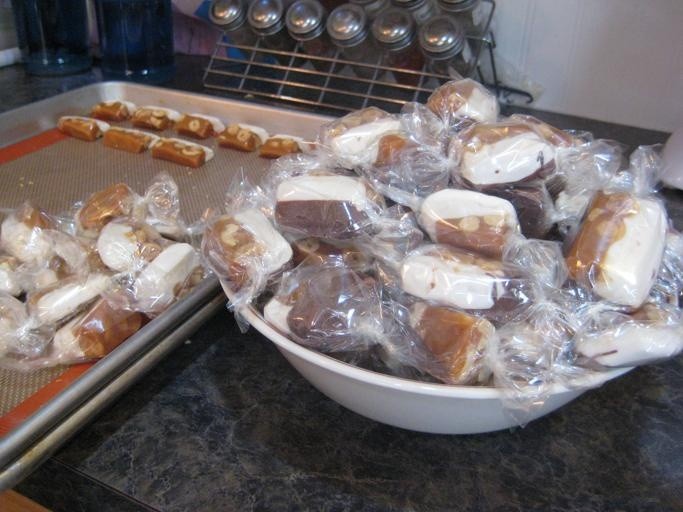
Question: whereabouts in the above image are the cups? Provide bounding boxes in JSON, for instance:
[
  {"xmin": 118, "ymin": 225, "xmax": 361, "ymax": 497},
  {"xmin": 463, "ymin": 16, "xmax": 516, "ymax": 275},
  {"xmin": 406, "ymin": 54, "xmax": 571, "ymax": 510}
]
[{"xmin": 11, "ymin": 0, "xmax": 175, "ymax": 78}]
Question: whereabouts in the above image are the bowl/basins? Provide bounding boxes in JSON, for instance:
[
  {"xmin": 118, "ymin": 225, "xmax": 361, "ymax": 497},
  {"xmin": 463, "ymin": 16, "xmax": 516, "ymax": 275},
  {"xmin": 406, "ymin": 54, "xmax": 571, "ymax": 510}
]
[{"xmin": 217, "ymin": 260, "xmax": 679, "ymax": 435}]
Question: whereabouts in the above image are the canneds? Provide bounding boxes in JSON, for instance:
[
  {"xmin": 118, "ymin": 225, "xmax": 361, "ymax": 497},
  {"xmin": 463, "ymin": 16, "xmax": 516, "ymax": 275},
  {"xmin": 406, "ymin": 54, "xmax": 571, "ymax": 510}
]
[{"xmin": 207, "ymin": 0, "xmax": 484, "ymax": 86}]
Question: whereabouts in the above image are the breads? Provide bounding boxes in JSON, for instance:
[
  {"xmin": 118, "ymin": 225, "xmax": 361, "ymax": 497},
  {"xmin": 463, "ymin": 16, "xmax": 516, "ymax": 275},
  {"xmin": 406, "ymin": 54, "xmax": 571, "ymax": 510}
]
[{"xmin": 0, "ymin": 78, "xmax": 668, "ymax": 384}]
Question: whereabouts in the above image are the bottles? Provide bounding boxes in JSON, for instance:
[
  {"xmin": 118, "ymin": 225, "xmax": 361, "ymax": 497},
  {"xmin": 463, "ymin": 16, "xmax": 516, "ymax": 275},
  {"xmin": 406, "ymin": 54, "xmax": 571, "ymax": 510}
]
[{"xmin": 209, "ymin": 0, "xmax": 479, "ymax": 89}]
